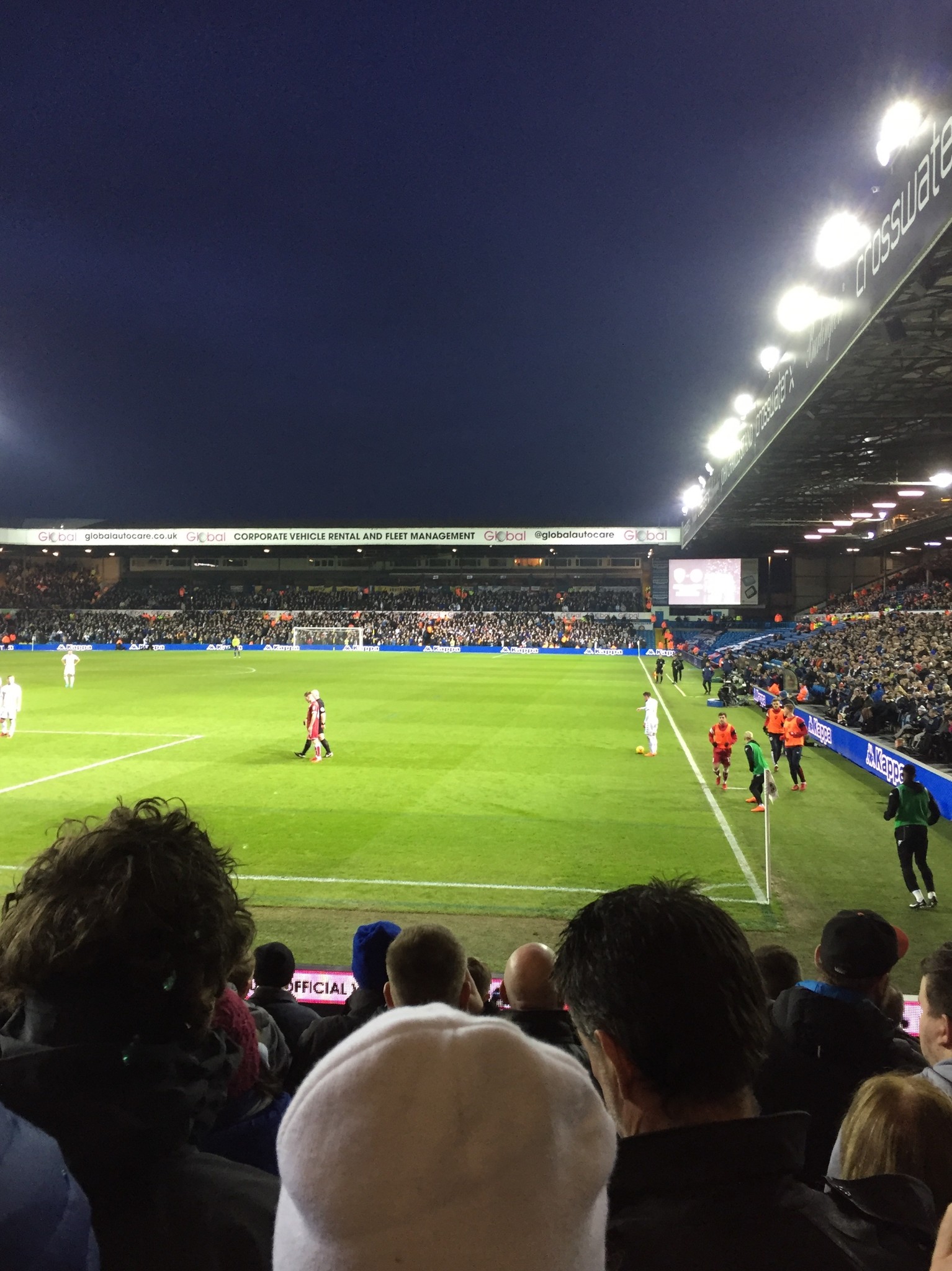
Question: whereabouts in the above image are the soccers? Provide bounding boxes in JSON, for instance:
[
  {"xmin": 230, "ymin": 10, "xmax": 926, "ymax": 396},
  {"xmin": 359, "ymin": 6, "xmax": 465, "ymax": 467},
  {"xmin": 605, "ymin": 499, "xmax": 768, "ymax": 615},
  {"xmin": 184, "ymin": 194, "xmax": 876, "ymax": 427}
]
[{"xmin": 636, "ymin": 745, "xmax": 644, "ymax": 754}]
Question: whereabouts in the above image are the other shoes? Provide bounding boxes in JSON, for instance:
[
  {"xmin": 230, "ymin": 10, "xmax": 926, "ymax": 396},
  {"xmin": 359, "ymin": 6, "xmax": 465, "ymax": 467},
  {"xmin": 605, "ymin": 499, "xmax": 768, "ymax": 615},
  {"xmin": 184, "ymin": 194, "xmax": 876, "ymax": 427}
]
[
  {"xmin": 705, "ymin": 691, "xmax": 708, "ymax": 694},
  {"xmin": 66, "ymin": 684, "xmax": 73, "ymax": 688},
  {"xmin": 1, "ymin": 732, "xmax": 14, "ymax": 739},
  {"xmin": 655, "ymin": 680, "xmax": 682, "ymax": 684},
  {"xmin": 645, "ymin": 753, "xmax": 657, "ymax": 757},
  {"xmin": 238, "ymin": 656, "xmax": 241, "ymax": 658},
  {"xmin": 234, "ymin": 656, "xmax": 236, "ymax": 658},
  {"xmin": 708, "ymin": 692, "xmax": 710, "ymax": 694}
]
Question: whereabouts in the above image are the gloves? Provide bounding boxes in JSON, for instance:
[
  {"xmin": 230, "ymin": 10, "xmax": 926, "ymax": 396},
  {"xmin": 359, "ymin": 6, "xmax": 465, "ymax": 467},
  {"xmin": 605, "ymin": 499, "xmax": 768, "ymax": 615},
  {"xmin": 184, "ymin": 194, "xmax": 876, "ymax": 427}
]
[
  {"xmin": 712, "ymin": 742, "xmax": 717, "ymax": 747},
  {"xmin": 779, "ymin": 736, "xmax": 784, "ymax": 741},
  {"xmin": 724, "ymin": 742, "xmax": 729, "ymax": 747},
  {"xmin": 763, "ymin": 726, "xmax": 767, "ymax": 731},
  {"xmin": 750, "ymin": 769, "xmax": 754, "ymax": 772},
  {"xmin": 789, "ymin": 731, "xmax": 796, "ymax": 737}
]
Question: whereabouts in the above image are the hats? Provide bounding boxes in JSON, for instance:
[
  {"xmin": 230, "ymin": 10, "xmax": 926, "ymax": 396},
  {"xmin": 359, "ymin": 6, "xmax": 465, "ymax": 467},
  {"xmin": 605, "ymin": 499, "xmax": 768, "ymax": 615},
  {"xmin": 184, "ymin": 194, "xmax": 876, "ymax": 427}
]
[
  {"xmin": 820, "ymin": 908, "xmax": 908, "ymax": 980},
  {"xmin": 918, "ymin": 705, "xmax": 926, "ymax": 711},
  {"xmin": 269, "ymin": 1003, "xmax": 617, "ymax": 1270},
  {"xmin": 351, "ymin": 922, "xmax": 401, "ymax": 990},
  {"xmin": 253, "ymin": 942, "xmax": 296, "ymax": 987}
]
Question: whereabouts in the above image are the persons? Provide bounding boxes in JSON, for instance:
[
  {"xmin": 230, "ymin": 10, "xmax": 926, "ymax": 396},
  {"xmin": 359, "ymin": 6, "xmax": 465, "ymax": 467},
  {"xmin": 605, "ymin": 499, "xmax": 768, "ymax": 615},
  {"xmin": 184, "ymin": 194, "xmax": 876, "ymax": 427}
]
[
  {"xmin": 709, "ymin": 713, "xmax": 737, "ymax": 789},
  {"xmin": 745, "ymin": 731, "xmax": 769, "ymax": 811},
  {"xmin": 779, "ymin": 704, "xmax": 808, "ymax": 791},
  {"xmin": 0, "ymin": 676, "xmax": 22, "ymax": 737},
  {"xmin": 0, "ymin": 559, "xmax": 657, "ymax": 659},
  {"xmin": 294, "ymin": 689, "xmax": 333, "ymax": 761},
  {"xmin": 884, "ymin": 763, "xmax": 940, "ymax": 908},
  {"xmin": 61, "ymin": 650, "xmax": 80, "ymax": 688},
  {"xmin": 636, "ymin": 692, "xmax": 659, "ymax": 756},
  {"xmin": 655, "ymin": 555, "xmax": 952, "ymax": 773},
  {"xmin": 0, "ymin": 798, "xmax": 952, "ymax": 1271}
]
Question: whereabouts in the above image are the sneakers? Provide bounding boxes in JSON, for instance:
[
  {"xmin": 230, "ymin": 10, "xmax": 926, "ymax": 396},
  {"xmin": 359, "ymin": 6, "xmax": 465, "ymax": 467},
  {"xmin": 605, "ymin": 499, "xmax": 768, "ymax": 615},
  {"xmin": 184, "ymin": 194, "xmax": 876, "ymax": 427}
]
[
  {"xmin": 715, "ymin": 776, "xmax": 764, "ymax": 812},
  {"xmin": 791, "ymin": 785, "xmax": 799, "ymax": 791},
  {"xmin": 909, "ymin": 899, "xmax": 926, "ymax": 909},
  {"xmin": 800, "ymin": 783, "xmax": 806, "ymax": 791},
  {"xmin": 774, "ymin": 766, "xmax": 779, "ymax": 772},
  {"xmin": 926, "ymin": 896, "xmax": 938, "ymax": 908},
  {"xmin": 294, "ymin": 751, "xmax": 334, "ymax": 762}
]
